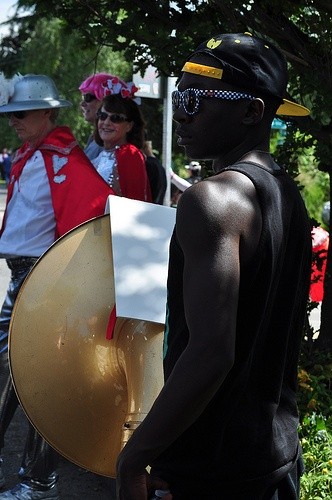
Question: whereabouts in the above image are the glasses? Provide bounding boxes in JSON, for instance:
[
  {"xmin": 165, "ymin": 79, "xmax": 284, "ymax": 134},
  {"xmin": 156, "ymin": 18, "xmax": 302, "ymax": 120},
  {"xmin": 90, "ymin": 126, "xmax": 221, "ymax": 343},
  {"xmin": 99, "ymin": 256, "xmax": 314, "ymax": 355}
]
[
  {"xmin": 172, "ymin": 88, "xmax": 255, "ymax": 116},
  {"xmin": 96, "ymin": 111, "xmax": 128, "ymax": 123},
  {"xmin": 82, "ymin": 93, "xmax": 96, "ymax": 102},
  {"xmin": 6, "ymin": 111, "xmax": 38, "ymax": 119}
]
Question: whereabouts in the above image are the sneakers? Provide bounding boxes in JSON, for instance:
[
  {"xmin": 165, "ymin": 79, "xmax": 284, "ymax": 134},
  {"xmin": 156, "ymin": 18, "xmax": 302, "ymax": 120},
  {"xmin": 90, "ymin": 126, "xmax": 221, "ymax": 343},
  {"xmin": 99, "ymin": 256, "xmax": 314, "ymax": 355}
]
[{"xmin": 0, "ymin": 473, "xmax": 59, "ymax": 500}]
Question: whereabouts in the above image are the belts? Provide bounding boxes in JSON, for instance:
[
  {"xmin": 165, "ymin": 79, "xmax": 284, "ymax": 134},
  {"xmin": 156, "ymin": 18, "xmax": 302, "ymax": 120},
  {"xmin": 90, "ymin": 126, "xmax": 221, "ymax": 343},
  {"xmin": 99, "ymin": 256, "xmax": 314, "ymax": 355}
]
[{"xmin": 6, "ymin": 258, "xmax": 37, "ymax": 269}]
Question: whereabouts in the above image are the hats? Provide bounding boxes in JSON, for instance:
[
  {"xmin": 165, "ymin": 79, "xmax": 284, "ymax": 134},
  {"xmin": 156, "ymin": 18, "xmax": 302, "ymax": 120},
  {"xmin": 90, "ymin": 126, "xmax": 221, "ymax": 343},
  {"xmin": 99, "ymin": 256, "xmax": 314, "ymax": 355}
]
[
  {"xmin": 0, "ymin": 74, "xmax": 72, "ymax": 113},
  {"xmin": 181, "ymin": 32, "xmax": 311, "ymax": 116},
  {"xmin": 184, "ymin": 161, "xmax": 201, "ymax": 170}
]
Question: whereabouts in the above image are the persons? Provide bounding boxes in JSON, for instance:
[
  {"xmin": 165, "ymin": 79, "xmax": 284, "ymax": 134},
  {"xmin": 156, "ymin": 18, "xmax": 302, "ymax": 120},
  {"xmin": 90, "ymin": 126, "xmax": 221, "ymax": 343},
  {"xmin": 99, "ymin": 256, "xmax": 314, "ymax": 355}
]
[
  {"xmin": 113, "ymin": 34, "xmax": 312, "ymax": 500},
  {"xmin": 0, "ymin": 74, "xmax": 332, "ymax": 500}
]
